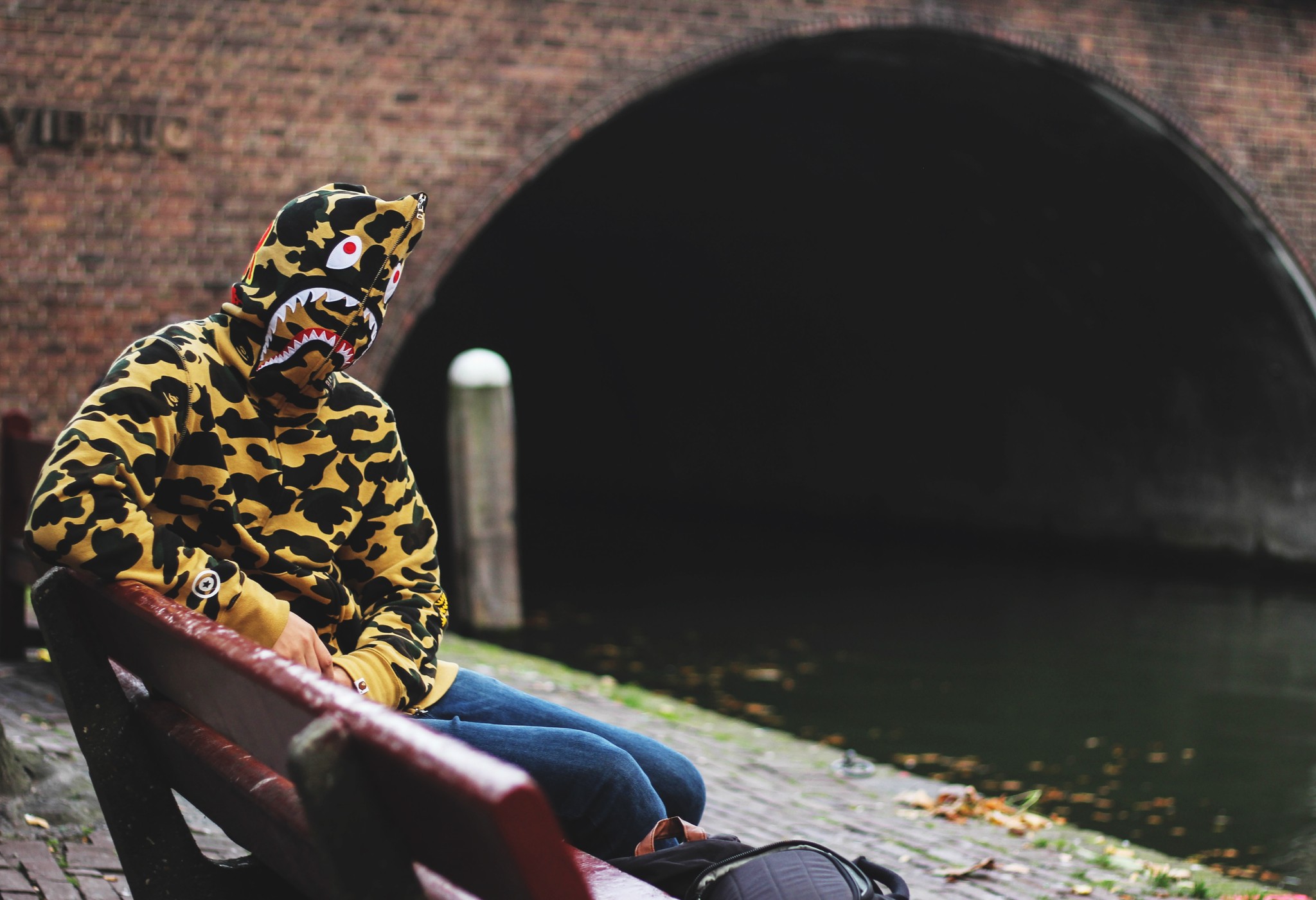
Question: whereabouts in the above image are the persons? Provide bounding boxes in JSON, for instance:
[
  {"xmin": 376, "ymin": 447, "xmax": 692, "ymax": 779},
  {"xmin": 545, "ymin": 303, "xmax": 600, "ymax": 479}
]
[{"xmin": 23, "ymin": 182, "xmax": 710, "ymax": 866}]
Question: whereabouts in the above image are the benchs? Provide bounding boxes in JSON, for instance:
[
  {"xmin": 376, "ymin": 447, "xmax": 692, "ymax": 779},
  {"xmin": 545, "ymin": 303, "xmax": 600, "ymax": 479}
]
[{"xmin": 26, "ymin": 569, "xmax": 688, "ymax": 899}]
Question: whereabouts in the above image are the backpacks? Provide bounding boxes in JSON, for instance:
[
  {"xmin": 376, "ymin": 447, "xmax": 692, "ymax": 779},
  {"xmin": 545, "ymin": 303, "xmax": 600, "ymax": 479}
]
[{"xmin": 606, "ymin": 816, "xmax": 910, "ymax": 900}]
